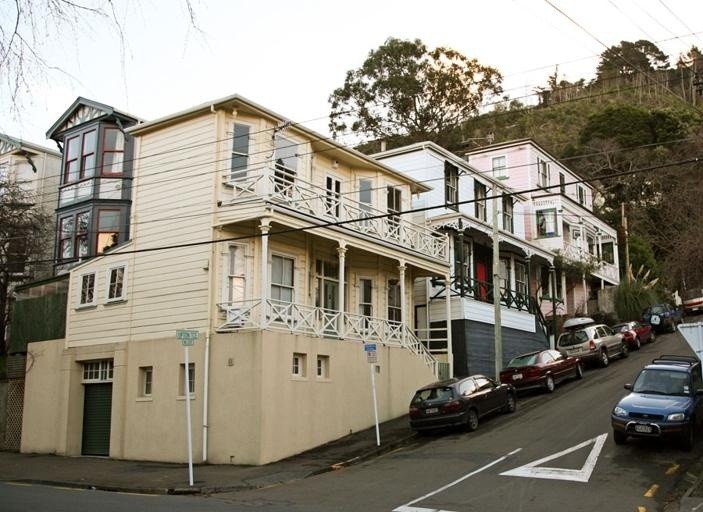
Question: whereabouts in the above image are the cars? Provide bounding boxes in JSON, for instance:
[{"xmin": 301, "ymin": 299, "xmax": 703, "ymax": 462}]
[
  {"xmin": 498, "ymin": 349, "xmax": 585, "ymax": 394},
  {"xmin": 406, "ymin": 374, "xmax": 519, "ymax": 433},
  {"xmin": 611, "ymin": 321, "xmax": 655, "ymax": 349}
]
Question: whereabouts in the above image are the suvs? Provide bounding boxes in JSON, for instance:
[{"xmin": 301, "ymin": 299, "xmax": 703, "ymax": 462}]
[
  {"xmin": 611, "ymin": 355, "xmax": 702, "ymax": 451},
  {"xmin": 557, "ymin": 316, "xmax": 628, "ymax": 368},
  {"xmin": 640, "ymin": 303, "xmax": 684, "ymax": 334}
]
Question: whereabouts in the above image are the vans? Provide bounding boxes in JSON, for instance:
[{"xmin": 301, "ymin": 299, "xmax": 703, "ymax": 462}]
[{"xmin": 682, "ymin": 288, "xmax": 702, "ymax": 316}]
